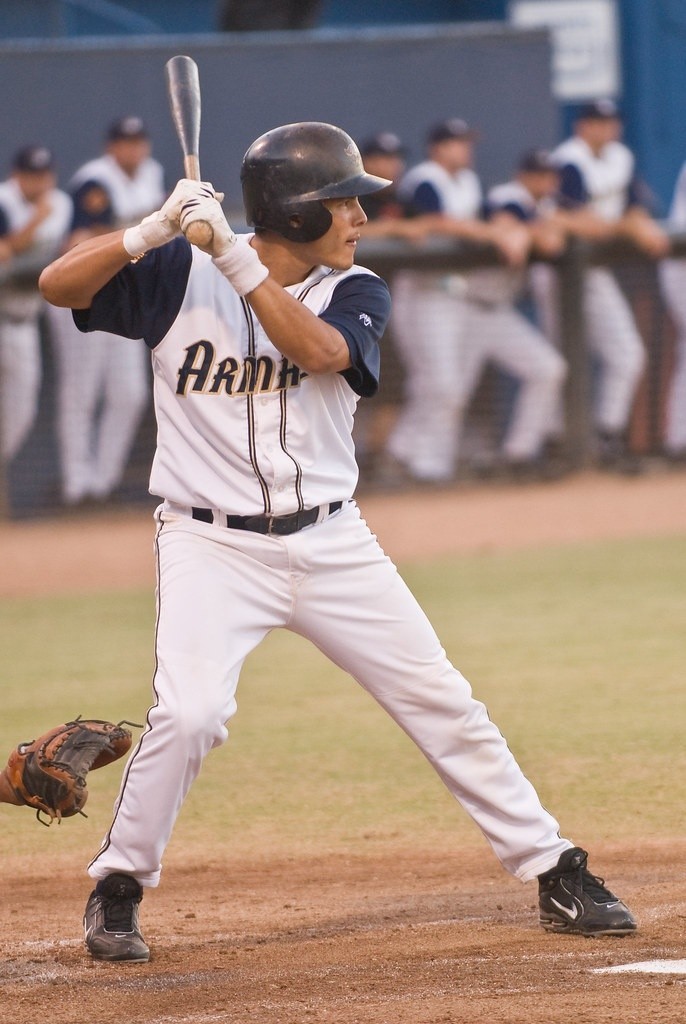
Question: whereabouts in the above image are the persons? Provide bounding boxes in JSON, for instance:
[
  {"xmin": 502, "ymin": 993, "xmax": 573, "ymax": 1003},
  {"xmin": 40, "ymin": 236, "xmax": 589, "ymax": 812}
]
[
  {"xmin": 0, "ymin": 92, "xmax": 686, "ymax": 522},
  {"xmin": 0, "ymin": 768, "xmax": 25, "ymax": 807},
  {"xmin": 38, "ymin": 121, "xmax": 639, "ymax": 960}
]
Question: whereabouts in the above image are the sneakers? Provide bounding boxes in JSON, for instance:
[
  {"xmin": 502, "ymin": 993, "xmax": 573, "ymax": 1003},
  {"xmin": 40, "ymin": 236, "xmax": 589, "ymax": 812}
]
[
  {"xmin": 537, "ymin": 846, "xmax": 638, "ymax": 938},
  {"xmin": 82, "ymin": 872, "xmax": 151, "ymax": 963}
]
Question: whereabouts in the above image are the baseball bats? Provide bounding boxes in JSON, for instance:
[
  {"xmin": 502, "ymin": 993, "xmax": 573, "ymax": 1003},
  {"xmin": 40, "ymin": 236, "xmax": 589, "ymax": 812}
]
[{"xmin": 166, "ymin": 53, "xmax": 216, "ymax": 251}]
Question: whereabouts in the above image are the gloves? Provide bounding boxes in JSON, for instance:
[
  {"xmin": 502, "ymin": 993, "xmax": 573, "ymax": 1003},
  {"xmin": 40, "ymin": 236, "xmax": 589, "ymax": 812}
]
[
  {"xmin": 122, "ymin": 178, "xmax": 225, "ymax": 257},
  {"xmin": 178, "ymin": 195, "xmax": 270, "ymax": 296}
]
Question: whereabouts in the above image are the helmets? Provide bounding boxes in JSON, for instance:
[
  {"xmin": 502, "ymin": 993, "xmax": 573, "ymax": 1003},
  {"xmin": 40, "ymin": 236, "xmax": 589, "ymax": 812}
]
[{"xmin": 240, "ymin": 121, "xmax": 394, "ymax": 243}]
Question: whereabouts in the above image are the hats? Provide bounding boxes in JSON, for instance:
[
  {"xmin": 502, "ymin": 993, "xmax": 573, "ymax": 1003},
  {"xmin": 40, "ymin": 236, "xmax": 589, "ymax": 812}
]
[
  {"xmin": 107, "ymin": 116, "xmax": 151, "ymax": 140},
  {"xmin": 360, "ymin": 128, "xmax": 410, "ymax": 159},
  {"xmin": 523, "ymin": 148, "xmax": 568, "ymax": 172},
  {"xmin": 574, "ymin": 97, "xmax": 625, "ymax": 120},
  {"xmin": 425, "ymin": 116, "xmax": 479, "ymax": 144},
  {"xmin": 13, "ymin": 143, "xmax": 59, "ymax": 174}
]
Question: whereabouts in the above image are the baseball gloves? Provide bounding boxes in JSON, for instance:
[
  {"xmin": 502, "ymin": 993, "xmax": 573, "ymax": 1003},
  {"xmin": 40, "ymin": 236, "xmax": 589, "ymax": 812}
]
[{"xmin": 6, "ymin": 718, "xmax": 133, "ymax": 823}]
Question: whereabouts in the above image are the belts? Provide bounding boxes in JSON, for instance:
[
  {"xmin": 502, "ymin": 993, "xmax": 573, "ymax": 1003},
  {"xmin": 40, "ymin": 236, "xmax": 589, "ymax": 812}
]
[
  {"xmin": 0, "ymin": 311, "xmax": 26, "ymax": 324},
  {"xmin": 192, "ymin": 499, "xmax": 352, "ymax": 535}
]
